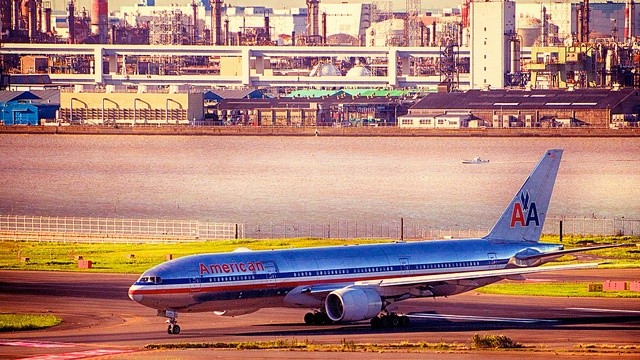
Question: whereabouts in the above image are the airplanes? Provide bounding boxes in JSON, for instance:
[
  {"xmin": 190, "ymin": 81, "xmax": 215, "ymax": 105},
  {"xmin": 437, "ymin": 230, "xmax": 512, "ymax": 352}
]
[{"xmin": 128, "ymin": 149, "xmax": 635, "ymax": 333}]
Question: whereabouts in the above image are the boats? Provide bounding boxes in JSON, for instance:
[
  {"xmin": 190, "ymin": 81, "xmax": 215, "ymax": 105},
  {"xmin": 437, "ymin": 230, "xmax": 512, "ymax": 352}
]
[{"xmin": 461, "ymin": 159, "xmax": 489, "ymax": 163}]
